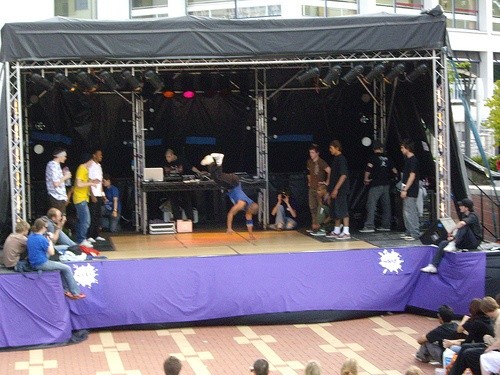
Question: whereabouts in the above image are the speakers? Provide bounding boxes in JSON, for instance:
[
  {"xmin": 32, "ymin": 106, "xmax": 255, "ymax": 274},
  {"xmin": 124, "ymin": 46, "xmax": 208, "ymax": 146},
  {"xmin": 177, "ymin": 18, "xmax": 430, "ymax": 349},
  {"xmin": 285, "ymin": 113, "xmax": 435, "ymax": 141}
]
[{"xmin": 419, "ymin": 217, "xmax": 455, "ymax": 245}]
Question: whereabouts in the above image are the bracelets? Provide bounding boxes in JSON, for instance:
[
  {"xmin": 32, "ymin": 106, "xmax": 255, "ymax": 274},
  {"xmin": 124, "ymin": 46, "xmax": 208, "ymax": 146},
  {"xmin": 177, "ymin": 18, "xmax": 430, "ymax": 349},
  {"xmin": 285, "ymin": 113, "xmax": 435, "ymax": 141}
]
[
  {"xmin": 113, "ymin": 209, "xmax": 117, "ymax": 212},
  {"xmin": 402, "ymin": 189, "xmax": 407, "ymax": 192}
]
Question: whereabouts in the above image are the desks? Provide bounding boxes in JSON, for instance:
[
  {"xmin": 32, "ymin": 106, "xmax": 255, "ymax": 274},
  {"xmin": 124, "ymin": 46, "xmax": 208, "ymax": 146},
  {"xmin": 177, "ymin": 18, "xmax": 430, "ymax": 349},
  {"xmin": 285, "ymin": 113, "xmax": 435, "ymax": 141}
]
[{"xmin": 140, "ymin": 177, "xmax": 267, "ymax": 236}]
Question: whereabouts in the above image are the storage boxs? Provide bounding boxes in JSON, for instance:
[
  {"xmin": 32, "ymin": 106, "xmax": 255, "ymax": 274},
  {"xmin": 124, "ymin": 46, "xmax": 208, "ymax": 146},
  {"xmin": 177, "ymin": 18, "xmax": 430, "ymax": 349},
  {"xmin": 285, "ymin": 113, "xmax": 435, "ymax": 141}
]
[
  {"xmin": 442, "ymin": 347, "xmax": 456, "ymax": 369},
  {"xmin": 149, "ymin": 220, "xmax": 176, "ymax": 235},
  {"xmin": 176, "ymin": 219, "xmax": 193, "ymax": 233}
]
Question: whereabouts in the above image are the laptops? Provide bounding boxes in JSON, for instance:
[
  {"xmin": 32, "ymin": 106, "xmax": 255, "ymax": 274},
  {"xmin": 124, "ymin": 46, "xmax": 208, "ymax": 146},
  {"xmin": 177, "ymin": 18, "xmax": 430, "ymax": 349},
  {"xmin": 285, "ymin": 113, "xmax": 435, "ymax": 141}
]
[{"xmin": 143, "ymin": 168, "xmax": 164, "ymax": 181}]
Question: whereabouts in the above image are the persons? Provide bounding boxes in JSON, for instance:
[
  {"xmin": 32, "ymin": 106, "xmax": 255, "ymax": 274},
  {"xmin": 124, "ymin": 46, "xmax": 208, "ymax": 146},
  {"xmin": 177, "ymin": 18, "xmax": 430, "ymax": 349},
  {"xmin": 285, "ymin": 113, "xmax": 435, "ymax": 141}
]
[
  {"xmin": 162, "ymin": 296, "xmax": 500, "ymax": 375},
  {"xmin": 1, "ymin": 139, "xmax": 420, "ymax": 300},
  {"xmin": 420, "ymin": 199, "xmax": 482, "ymax": 273}
]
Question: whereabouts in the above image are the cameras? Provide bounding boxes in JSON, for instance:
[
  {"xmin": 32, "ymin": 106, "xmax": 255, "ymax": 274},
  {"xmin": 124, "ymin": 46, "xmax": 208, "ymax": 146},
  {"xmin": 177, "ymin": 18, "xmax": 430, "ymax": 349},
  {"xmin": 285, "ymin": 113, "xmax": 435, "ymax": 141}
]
[{"xmin": 281, "ymin": 194, "xmax": 285, "ymax": 199}]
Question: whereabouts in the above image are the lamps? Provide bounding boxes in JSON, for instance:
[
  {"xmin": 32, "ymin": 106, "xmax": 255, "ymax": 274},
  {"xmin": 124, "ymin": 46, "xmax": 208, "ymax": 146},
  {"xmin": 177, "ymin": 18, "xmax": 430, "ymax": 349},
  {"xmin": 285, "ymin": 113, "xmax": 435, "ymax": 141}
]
[
  {"xmin": 203, "ymin": 73, "xmax": 218, "ymax": 99},
  {"xmin": 99, "ymin": 70, "xmax": 120, "ymax": 92},
  {"xmin": 362, "ymin": 63, "xmax": 385, "ymax": 86},
  {"xmin": 294, "ymin": 65, "xmax": 319, "ymax": 87},
  {"xmin": 161, "ymin": 74, "xmax": 175, "ymax": 98},
  {"xmin": 219, "ymin": 72, "xmax": 234, "ymax": 96},
  {"xmin": 341, "ymin": 65, "xmax": 365, "ymax": 87},
  {"xmin": 144, "ymin": 70, "xmax": 164, "ymax": 94},
  {"xmin": 51, "ymin": 72, "xmax": 76, "ymax": 95},
  {"xmin": 382, "ymin": 63, "xmax": 405, "ymax": 85},
  {"xmin": 182, "ymin": 75, "xmax": 195, "ymax": 99},
  {"xmin": 76, "ymin": 71, "xmax": 99, "ymax": 93},
  {"xmin": 119, "ymin": 70, "xmax": 142, "ymax": 92},
  {"xmin": 403, "ymin": 63, "xmax": 429, "ymax": 84},
  {"xmin": 322, "ymin": 65, "xmax": 342, "ymax": 88},
  {"xmin": 238, "ymin": 68, "xmax": 252, "ymax": 96},
  {"xmin": 29, "ymin": 72, "xmax": 55, "ymax": 93}
]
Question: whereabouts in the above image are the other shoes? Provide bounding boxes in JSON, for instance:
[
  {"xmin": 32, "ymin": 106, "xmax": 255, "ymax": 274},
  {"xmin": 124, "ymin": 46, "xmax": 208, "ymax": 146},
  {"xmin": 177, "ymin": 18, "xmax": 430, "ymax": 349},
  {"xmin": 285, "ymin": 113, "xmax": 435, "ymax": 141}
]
[
  {"xmin": 358, "ymin": 227, "xmax": 375, "ymax": 232},
  {"xmin": 399, "ymin": 233, "xmax": 417, "ymax": 240},
  {"xmin": 335, "ymin": 231, "xmax": 351, "ymax": 240},
  {"xmin": 88, "ymin": 237, "xmax": 96, "ymax": 243},
  {"xmin": 442, "ymin": 241, "xmax": 456, "ymax": 252},
  {"xmin": 309, "ymin": 230, "xmax": 326, "ymax": 236},
  {"xmin": 200, "ymin": 155, "xmax": 214, "ymax": 166},
  {"xmin": 64, "ymin": 292, "xmax": 87, "ymax": 300},
  {"xmin": 211, "ymin": 152, "xmax": 225, "ymax": 164},
  {"xmin": 325, "ymin": 231, "xmax": 341, "ymax": 238},
  {"xmin": 375, "ymin": 226, "xmax": 391, "ymax": 231},
  {"xmin": 416, "ymin": 350, "xmax": 431, "ymax": 363},
  {"xmin": 420, "ymin": 264, "xmax": 438, "ymax": 273},
  {"xmin": 80, "ymin": 240, "xmax": 93, "ymax": 248},
  {"xmin": 96, "ymin": 236, "xmax": 106, "ymax": 240},
  {"xmin": 306, "ymin": 229, "xmax": 314, "ymax": 233}
]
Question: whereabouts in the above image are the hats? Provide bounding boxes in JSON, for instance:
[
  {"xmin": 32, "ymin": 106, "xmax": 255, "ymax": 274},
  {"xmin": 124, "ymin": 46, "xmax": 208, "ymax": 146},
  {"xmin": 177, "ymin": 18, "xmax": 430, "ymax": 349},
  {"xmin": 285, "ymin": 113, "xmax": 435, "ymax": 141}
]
[
  {"xmin": 457, "ymin": 198, "xmax": 473, "ymax": 208},
  {"xmin": 440, "ymin": 305, "xmax": 453, "ymax": 320},
  {"xmin": 53, "ymin": 148, "xmax": 66, "ymax": 157},
  {"xmin": 331, "ymin": 139, "xmax": 343, "ymax": 151}
]
